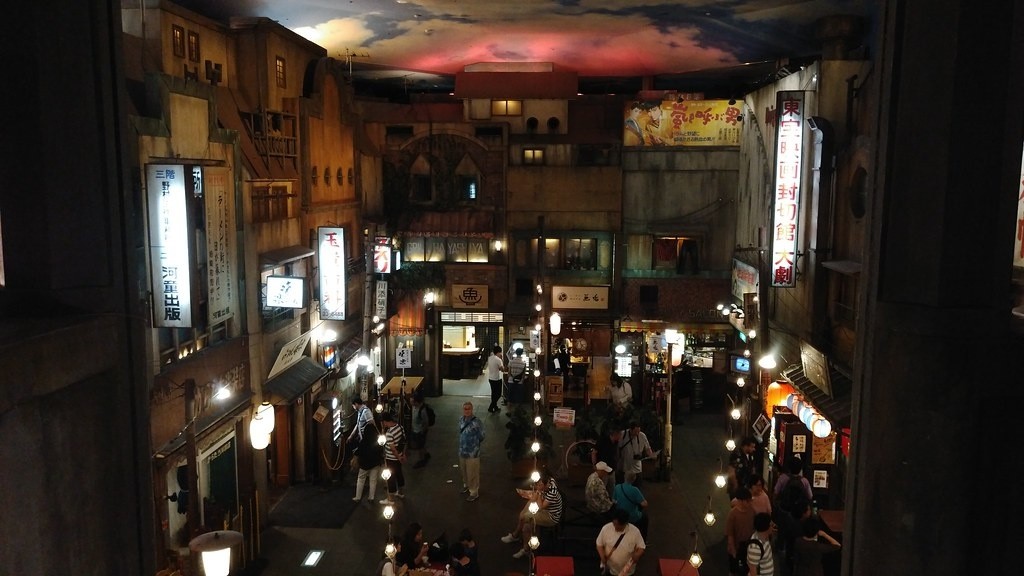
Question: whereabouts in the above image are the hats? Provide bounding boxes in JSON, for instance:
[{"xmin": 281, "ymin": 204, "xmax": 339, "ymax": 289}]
[{"xmin": 596, "ymin": 461, "xmax": 613, "ymax": 472}]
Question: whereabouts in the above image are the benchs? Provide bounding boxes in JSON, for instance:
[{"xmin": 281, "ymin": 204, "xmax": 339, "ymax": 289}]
[{"xmin": 534, "ymin": 490, "xmax": 613, "ymax": 543}]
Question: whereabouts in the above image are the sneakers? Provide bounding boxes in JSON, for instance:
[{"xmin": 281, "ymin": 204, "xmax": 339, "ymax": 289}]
[
  {"xmin": 513, "ymin": 549, "xmax": 530, "ymax": 559},
  {"xmin": 501, "ymin": 533, "xmax": 519, "ymax": 543}
]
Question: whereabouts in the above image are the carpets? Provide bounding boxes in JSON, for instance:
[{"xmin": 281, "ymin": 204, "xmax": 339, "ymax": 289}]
[{"xmin": 268, "ymin": 477, "xmax": 369, "ymax": 529}]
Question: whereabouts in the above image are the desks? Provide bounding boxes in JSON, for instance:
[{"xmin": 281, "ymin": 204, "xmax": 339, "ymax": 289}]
[
  {"xmin": 533, "ymin": 556, "xmax": 575, "ymax": 576},
  {"xmin": 380, "ymin": 374, "xmax": 425, "ymax": 411},
  {"xmin": 817, "ymin": 509, "xmax": 845, "ymax": 533},
  {"xmin": 411, "ymin": 560, "xmax": 456, "ymax": 576},
  {"xmin": 658, "ymin": 556, "xmax": 703, "ymax": 575}
]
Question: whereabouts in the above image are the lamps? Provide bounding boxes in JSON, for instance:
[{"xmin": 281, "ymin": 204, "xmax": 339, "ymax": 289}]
[
  {"xmin": 728, "ymin": 95, "xmax": 737, "ymax": 106},
  {"xmin": 737, "ymin": 113, "xmax": 745, "ymax": 122},
  {"xmin": 677, "ymin": 94, "xmax": 684, "ymax": 104}
]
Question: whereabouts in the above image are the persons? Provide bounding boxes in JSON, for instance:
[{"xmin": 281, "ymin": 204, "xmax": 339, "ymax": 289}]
[
  {"xmin": 381, "ymin": 413, "xmax": 408, "ymax": 499},
  {"xmin": 376, "ymin": 523, "xmax": 480, "ymax": 576},
  {"xmin": 501, "ymin": 469, "xmax": 562, "ymax": 559},
  {"xmin": 785, "ymin": 500, "xmax": 811, "ymax": 566},
  {"xmin": 483, "ymin": 346, "xmax": 504, "ymax": 413},
  {"xmin": 504, "ymin": 407, "xmax": 532, "ymax": 470},
  {"xmin": 559, "ymin": 345, "xmax": 570, "ymax": 391},
  {"xmin": 596, "ymin": 509, "xmax": 646, "ymax": 576},
  {"xmin": 791, "ymin": 519, "xmax": 841, "ymax": 576},
  {"xmin": 506, "ymin": 348, "xmax": 527, "ymax": 417},
  {"xmin": 352, "ymin": 423, "xmax": 385, "ymax": 500},
  {"xmin": 458, "ymin": 403, "xmax": 485, "ymax": 501},
  {"xmin": 570, "ymin": 422, "xmax": 657, "ymax": 548},
  {"xmin": 727, "ymin": 488, "xmax": 777, "ymax": 576},
  {"xmin": 410, "ymin": 394, "xmax": 428, "ymax": 468},
  {"xmin": 609, "ymin": 373, "xmax": 633, "ymax": 417},
  {"xmin": 773, "ymin": 457, "xmax": 813, "ymax": 552},
  {"xmin": 727, "ymin": 438, "xmax": 755, "ymax": 501},
  {"xmin": 345, "ymin": 398, "xmax": 375, "ymax": 444},
  {"xmin": 747, "ymin": 512, "xmax": 774, "ymax": 576},
  {"xmin": 748, "ymin": 475, "xmax": 772, "ymax": 518}
]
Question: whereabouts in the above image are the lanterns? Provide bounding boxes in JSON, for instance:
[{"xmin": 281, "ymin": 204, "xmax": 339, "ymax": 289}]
[
  {"xmin": 766, "ymin": 380, "xmax": 831, "ymax": 438},
  {"xmin": 550, "ymin": 312, "xmax": 561, "ymax": 335},
  {"xmin": 672, "ymin": 343, "xmax": 681, "ymax": 366},
  {"xmin": 248, "ymin": 401, "xmax": 275, "ymax": 450}
]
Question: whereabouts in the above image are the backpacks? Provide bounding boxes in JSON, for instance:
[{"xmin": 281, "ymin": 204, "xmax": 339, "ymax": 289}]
[
  {"xmin": 418, "ymin": 403, "xmax": 435, "ymax": 427},
  {"xmin": 735, "ymin": 539, "xmax": 764, "ymax": 576},
  {"xmin": 780, "ymin": 473, "xmax": 806, "ymax": 513}
]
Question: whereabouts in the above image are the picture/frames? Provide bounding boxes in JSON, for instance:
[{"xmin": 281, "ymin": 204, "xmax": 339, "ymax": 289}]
[{"xmin": 752, "ymin": 414, "xmax": 770, "ymax": 437}]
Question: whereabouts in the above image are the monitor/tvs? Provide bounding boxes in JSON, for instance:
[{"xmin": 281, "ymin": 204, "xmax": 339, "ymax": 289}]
[{"xmin": 731, "ymin": 356, "xmax": 752, "ymax": 375}]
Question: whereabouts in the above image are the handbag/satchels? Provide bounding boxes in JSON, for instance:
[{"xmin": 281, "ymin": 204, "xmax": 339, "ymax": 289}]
[{"xmin": 396, "ymin": 425, "xmax": 406, "ymax": 454}]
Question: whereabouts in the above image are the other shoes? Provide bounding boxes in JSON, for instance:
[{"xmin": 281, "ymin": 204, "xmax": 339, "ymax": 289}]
[
  {"xmin": 495, "ymin": 408, "xmax": 501, "ymax": 411},
  {"xmin": 488, "ymin": 409, "xmax": 495, "ymax": 413},
  {"xmin": 368, "ymin": 497, "xmax": 374, "ymax": 501},
  {"xmin": 424, "ymin": 452, "xmax": 431, "ymax": 462},
  {"xmin": 464, "ymin": 496, "xmax": 479, "ymax": 503},
  {"xmin": 413, "ymin": 460, "xmax": 425, "ymax": 468},
  {"xmin": 353, "ymin": 497, "xmax": 361, "ymax": 501},
  {"xmin": 394, "ymin": 491, "xmax": 404, "ymax": 498},
  {"xmin": 460, "ymin": 488, "xmax": 469, "ymax": 496},
  {"xmin": 387, "ymin": 493, "xmax": 393, "ymax": 502}
]
